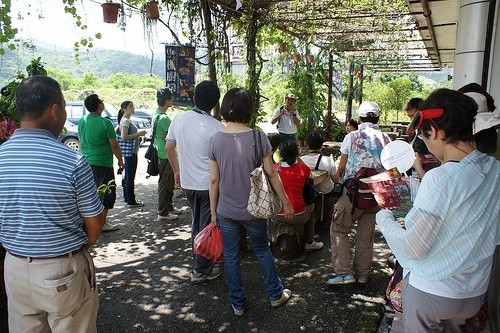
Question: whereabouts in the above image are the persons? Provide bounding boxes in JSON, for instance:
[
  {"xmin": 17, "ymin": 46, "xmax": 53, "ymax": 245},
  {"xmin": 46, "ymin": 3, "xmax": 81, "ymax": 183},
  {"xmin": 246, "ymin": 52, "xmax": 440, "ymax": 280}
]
[
  {"xmin": 115, "ymin": 101, "xmax": 148, "ymax": 208},
  {"xmin": 406, "ymin": 83, "xmax": 500, "ymax": 207},
  {"xmin": 164, "ymin": 80, "xmax": 251, "ymax": 283},
  {"xmin": 376, "ymin": 88, "xmax": 500, "ymax": 333},
  {"xmin": 259, "ymin": 119, "xmax": 358, "ymax": 253},
  {"xmin": 78, "ymin": 94, "xmax": 124, "ymax": 233},
  {"xmin": 271, "ymin": 92, "xmax": 302, "ymax": 151},
  {"xmin": 0, "ymin": 75, "xmax": 105, "ymax": 333},
  {"xmin": 208, "ymin": 87, "xmax": 295, "ymax": 316},
  {"xmin": 144, "ymin": 86, "xmax": 184, "ymax": 221},
  {"xmin": 326, "ymin": 101, "xmax": 392, "ymax": 284}
]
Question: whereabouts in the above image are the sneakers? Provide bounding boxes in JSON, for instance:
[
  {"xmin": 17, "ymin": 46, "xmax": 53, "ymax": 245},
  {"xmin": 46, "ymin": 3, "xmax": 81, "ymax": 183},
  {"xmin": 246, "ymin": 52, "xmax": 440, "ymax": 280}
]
[
  {"xmin": 356, "ymin": 275, "xmax": 368, "ymax": 284},
  {"xmin": 127, "ymin": 202, "xmax": 143, "ymax": 207},
  {"xmin": 158, "ymin": 213, "xmax": 179, "ymax": 219},
  {"xmin": 328, "ymin": 275, "xmax": 356, "ymax": 284},
  {"xmin": 190, "ymin": 268, "xmax": 221, "ymax": 283},
  {"xmin": 232, "ymin": 305, "xmax": 245, "ymax": 316},
  {"xmin": 305, "ymin": 241, "xmax": 323, "ymax": 251},
  {"xmin": 271, "ymin": 289, "xmax": 291, "ymax": 306},
  {"xmin": 169, "ymin": 208, "xmax": 183, "ymax": 213},
  {"xmin": 101, "ymin": 222, "xmax": 119, "ymax": 231}
]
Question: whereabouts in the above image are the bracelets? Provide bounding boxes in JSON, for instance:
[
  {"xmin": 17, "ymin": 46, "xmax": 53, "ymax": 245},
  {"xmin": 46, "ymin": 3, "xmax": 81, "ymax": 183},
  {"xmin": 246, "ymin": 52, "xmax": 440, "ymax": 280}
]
[
  {"xmin": 292, "ymin": 114, "xmax": 296, "ymax": 117},
  {"xmin": 210, "ymin": 211, "xmax": 217, "ymax": 216}
]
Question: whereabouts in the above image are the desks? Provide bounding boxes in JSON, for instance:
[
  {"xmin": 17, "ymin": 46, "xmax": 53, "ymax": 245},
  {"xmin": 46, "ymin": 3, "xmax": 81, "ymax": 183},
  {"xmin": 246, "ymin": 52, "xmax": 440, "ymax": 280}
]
[{"xmin": 306, "ymin": 168, "xmax": 330, "ymax": 200}]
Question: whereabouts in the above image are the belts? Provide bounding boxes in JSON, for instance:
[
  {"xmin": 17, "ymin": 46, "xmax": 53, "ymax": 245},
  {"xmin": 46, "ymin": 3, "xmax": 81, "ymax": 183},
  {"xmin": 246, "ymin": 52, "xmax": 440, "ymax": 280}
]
[{"xmin": 9, "ymin": 248, "xmax": 83, "ymax": 258}]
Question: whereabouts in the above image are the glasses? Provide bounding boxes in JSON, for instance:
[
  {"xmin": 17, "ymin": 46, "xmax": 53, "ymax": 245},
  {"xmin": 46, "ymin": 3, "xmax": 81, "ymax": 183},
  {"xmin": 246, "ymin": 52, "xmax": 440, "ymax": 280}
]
[
  {"xmin": 416, "ymin": 124, "xmax": 430, "ymax": 139},
  {"xmin": 99, "ymin": 100, "xmax": 105, "ymax": 103}
]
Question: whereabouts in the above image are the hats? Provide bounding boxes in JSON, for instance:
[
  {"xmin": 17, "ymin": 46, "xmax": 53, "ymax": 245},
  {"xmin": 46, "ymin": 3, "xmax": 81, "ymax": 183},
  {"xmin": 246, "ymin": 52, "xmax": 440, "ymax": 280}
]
[
  {"xmin": 359, "ymin": 102, "xmax": 380, "ymax": 117},
  {"xmin": 406, "ymin": 109, "xmax": 443, "ymax": 134},
  {"xmin": 157, "ymin": 87, "xmax": 172, "ymax": 98},
  {"xmin": 285, "ymin": 94, "xmax": 299, "ymax": 99},
  {"xmin": 463, "ymin": 92, "xmax": 500, "ymax": 136}
]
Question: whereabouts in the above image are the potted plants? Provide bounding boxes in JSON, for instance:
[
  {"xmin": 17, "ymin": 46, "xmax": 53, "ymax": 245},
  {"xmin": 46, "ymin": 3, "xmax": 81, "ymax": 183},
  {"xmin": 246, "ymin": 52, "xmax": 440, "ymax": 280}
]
[
  {"xmin": 98, "ymin": 179, "xmax": 118, "ymax": 208},
  {"xmin": 100, "ymin": 1, "xmax": 122, "ymax": 25},
  {"xmin": 306, "ymin": 52, "xmax": 315, "ymax": 63},
  {"xmin": 291, "ymin": 51, "xmax": 303, "ymax": 62},
  {"xmin": 352, "ymin": 68, "xmax": 371, "ymax": 81},
  {"xmin": 275, "ymin": 38, "xmax": 292, "ymax": 53},
  {"xmin": 140, "ymin": 1, "xmax": 163, "ymax": 22},
  {"xmin": 297, "ymin": 122, "xmax": 309, "ymax": 148}
]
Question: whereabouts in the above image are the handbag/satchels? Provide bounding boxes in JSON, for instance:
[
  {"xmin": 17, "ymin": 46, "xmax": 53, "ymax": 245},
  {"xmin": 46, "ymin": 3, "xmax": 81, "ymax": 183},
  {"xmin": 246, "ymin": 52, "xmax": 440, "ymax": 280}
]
[
  {"xmin": 246, "ymin": 130, "xmax": 283, "ymax": 218},
  {"xmin": 346, "ymin": 167, "xmax": 379, "ymax": 210},
  {"xmin": 302, "ymin": 178, "xmax": 318, "ymax": 204},
  {"xmin": 193, "ymin": 223, "xmax": 225, "ymax": 261},
  {"xmin": 385, "ymin": 266, "xmax": 404, "ymax": 312},
  {"xmin": 145, "ymin": 145, "xmax": 158, "ymax": 161}
]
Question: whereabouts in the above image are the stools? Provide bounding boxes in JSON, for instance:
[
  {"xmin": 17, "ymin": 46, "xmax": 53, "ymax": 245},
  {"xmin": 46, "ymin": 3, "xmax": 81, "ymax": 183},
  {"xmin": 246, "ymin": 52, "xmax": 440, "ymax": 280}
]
[{"xmin": 269, "ymin": 222, "xmax": 308, "ymax": 267}]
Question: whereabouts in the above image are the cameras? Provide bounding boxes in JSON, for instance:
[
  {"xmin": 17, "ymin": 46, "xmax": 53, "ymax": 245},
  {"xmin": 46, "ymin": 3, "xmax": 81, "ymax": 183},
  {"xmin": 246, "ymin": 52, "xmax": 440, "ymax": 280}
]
[{"xmin": 118, "ymin": 168, "xmax": 122, "ymax": 174}]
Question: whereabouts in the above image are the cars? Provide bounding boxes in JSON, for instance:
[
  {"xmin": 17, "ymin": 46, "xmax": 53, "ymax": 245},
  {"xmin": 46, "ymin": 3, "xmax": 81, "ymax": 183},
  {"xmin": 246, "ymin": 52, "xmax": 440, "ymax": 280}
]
[
  {"xmin": 132, "ymin": 109, "xmax": 153, "ymax": 124},
  {"xmin": 58, "ymin": 120, "xmax": 80, "ymax": 151}
]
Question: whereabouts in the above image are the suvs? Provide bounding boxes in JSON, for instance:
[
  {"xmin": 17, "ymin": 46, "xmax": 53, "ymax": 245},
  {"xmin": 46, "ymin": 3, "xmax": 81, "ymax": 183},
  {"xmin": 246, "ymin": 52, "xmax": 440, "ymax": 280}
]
[{"xmin": 65, "ymin": 101, "xmax": 153, "ymax": 147}]
